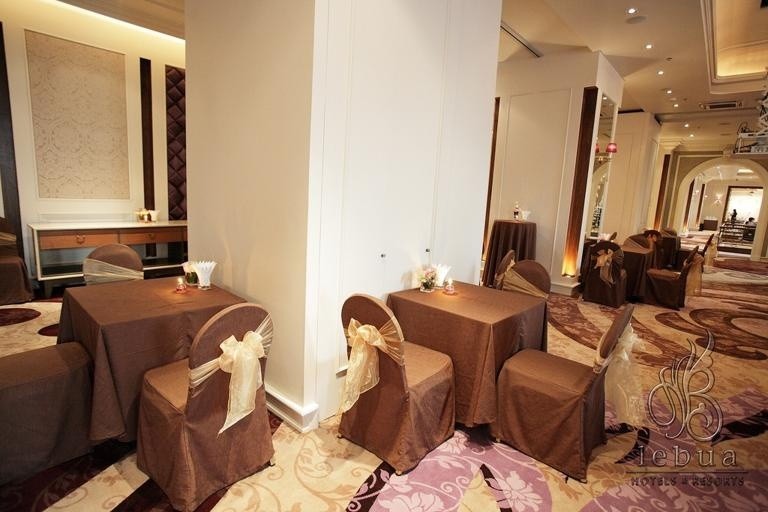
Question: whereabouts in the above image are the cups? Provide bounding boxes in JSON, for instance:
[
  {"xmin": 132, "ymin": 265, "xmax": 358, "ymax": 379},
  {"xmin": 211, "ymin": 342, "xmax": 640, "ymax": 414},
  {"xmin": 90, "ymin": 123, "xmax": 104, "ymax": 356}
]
[
  {"xmin": 182, "ymin": 260, "xmax": 218, "ymax": 290},
  {"xmin": 520, "ymin": 209, "xmax": 532, "ymax": 221},
  {"xmin": 435, "ymin": 263, "xmax": 452, "ymax": 290}
]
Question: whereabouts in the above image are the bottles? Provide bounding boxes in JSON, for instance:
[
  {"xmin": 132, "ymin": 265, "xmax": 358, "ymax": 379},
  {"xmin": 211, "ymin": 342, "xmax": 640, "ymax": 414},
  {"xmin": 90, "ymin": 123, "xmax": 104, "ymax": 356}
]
[
  {"xmin": 418, "ymin": 247, "xmax": 439, "ymax": 293},
  {"xmin": 513, "ymin": 201, "xmax": 520, "ymax": 220}
]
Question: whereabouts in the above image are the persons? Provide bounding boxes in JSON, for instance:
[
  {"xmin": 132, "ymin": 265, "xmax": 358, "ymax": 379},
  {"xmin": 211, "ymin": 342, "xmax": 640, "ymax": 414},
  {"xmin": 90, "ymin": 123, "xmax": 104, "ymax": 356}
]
[
  {"xmin": 728, "ymin": 208, "xmax": 737, "ymax": 228},
  {"xmin": 744, "ymin": 217, "xmax": 756, "ymax": 226}
]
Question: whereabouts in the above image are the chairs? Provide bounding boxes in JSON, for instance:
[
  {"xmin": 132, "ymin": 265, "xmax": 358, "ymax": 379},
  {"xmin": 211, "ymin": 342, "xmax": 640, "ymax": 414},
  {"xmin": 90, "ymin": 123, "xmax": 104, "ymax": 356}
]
[{"xmin": 1, "ymin": 220, "xmax": 718, "ymax": 512}]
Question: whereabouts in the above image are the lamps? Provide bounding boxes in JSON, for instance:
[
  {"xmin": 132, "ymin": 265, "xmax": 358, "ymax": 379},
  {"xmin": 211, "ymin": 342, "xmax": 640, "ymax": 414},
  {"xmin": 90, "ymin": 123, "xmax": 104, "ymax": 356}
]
[{"xmin": 594, "ymin": 143, "xmax": 618, "ymax": 164}]
